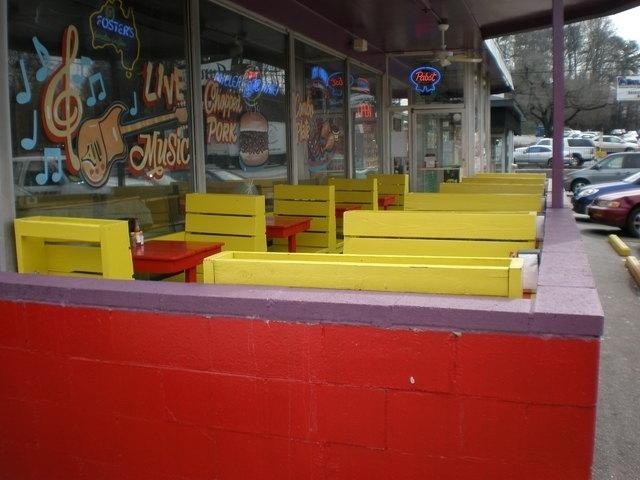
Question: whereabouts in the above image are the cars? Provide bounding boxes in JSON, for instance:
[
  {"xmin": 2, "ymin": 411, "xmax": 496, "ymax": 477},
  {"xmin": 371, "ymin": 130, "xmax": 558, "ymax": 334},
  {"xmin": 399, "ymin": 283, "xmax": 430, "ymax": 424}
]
[
  {"xmin": 572, "ymin": 171, "xmax": 640, "ymax": 214},
  {"xmin": 586, "ymin": 189, "xmax": 640, "ymax": 236},
  {"xmin": 513, "ymin": 128, "xmax": 639, "ymax": 169}
]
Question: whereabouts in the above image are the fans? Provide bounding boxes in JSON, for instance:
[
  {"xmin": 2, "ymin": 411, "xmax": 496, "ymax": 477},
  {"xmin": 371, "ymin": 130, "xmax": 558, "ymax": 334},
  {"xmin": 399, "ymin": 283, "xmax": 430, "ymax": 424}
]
[{"xmin": 419, "ymin": 22, "xmax": 484, "ymax": 68}]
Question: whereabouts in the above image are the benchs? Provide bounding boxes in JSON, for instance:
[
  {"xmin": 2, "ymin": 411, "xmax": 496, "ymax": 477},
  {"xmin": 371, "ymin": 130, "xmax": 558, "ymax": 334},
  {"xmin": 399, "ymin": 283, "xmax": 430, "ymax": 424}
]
[{"xmin": 12, "ymin": 166, "xmax": 549, "ymax": 300}]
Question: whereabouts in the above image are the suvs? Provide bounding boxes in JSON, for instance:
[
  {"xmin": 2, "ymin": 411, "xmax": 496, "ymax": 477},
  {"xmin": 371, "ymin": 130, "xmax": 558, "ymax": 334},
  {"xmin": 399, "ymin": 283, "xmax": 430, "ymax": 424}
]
[{"xmin": 564, "ymin": 152, "xmax": 640, "ymax": 193}]
